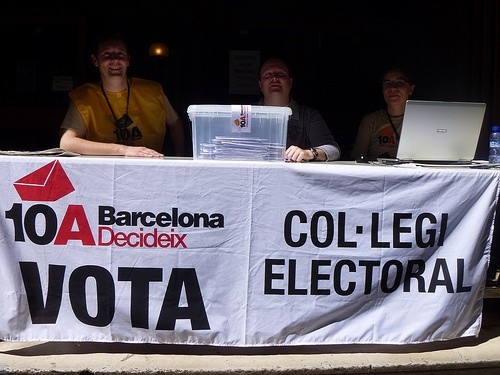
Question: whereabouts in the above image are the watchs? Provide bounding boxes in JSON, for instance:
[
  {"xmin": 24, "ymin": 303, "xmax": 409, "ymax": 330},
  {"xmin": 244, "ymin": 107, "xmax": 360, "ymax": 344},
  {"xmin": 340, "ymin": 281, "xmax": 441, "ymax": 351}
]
[{"xmin": 308, "ymin": 148, "xmax": 318, "ymax": 161}]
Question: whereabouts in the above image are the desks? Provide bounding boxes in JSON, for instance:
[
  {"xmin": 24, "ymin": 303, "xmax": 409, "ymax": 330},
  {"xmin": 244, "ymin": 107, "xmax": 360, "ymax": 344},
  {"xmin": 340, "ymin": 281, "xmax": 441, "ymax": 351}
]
[{"xmin": 0, "ymin": 155, "xmax": 500, "ymax": 348}]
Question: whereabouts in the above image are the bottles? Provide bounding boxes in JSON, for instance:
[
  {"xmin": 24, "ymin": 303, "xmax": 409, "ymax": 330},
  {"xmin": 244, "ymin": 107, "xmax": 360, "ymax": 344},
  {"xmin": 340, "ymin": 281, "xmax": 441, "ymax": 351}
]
[{"xmin": 489, "ymin": 126, "xmax": 500, "ymax": 164}]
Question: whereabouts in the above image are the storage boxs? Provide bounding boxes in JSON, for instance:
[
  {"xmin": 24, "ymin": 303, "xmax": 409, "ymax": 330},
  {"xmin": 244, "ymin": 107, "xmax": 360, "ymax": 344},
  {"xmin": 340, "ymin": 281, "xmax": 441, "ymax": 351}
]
[{"xmin": 187, "ymin": 103, "xmax": 292, "ymax": 162}]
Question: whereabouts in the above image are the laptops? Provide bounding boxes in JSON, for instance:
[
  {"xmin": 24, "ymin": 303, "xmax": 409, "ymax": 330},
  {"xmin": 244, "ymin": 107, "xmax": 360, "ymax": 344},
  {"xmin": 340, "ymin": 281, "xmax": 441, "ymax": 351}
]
[{"xmin": 377, "ymin": 100, "xmax": 486, "ymax": 164}]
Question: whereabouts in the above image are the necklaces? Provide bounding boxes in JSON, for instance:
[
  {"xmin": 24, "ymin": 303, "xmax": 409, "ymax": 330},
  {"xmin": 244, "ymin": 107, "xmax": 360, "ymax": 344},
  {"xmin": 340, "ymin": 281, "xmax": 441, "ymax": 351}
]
[
  {"xmin": 100, "ymin": 76, "xmax": 131, "ymax": 143},
  {"xmin": 389, "ymin": 115, "xmax": 400, "ymax": 139}
]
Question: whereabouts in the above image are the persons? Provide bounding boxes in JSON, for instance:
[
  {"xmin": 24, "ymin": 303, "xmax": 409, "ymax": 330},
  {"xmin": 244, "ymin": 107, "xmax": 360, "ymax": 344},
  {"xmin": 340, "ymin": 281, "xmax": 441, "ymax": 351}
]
[
  {"xmin": 253, "ymin": 58, "xmax": 340, "ymax": 162},
  {"xmin": 353, "ymin": 65, "xmax": 417, "ymax": 161},
  {"xmin": 57, "ymin": 32, "xmax": 185, "ymax": 158}
]
